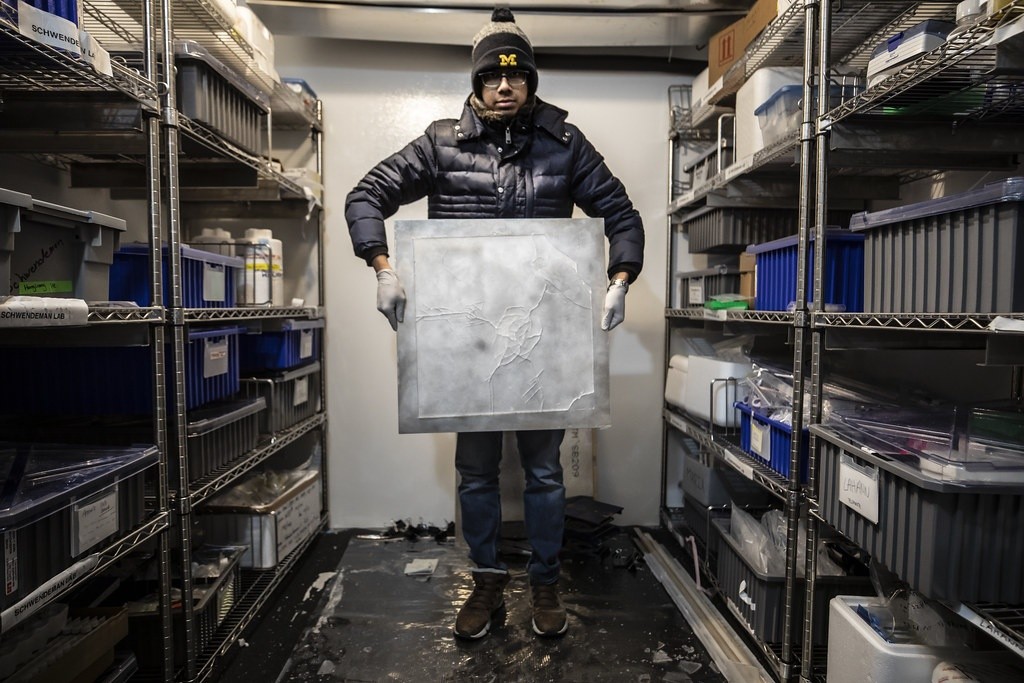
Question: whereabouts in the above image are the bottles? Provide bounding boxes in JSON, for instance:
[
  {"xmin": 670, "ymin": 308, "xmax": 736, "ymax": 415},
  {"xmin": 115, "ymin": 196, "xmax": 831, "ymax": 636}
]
[{"xmin": 193, "ymin": 228, "xmax": 283, "ymax": 306}]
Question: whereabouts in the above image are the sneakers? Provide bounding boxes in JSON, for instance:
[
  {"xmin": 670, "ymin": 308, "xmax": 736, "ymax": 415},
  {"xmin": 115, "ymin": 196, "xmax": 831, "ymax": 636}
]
[
  {"xmin": 454, "ymin": 566, "xmax": 511, "ymax": 639},
  {"xmin": 529, "ymin": 581, "xmax": 568, "ymax": 636}
]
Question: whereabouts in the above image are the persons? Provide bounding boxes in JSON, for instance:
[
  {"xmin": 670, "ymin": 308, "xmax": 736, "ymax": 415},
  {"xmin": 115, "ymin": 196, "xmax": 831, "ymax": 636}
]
[{"xmin": 345, "ymin": 9, "xmax": 645, "ymax": 640}]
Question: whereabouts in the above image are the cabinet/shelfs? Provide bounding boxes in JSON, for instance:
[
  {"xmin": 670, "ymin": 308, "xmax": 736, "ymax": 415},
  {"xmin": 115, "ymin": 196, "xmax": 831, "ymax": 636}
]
[
  {"xmin": 796, "ymin": 0, "xmax": 1024, "ymax": 683},
  {"xmin": 658, "ymin": 0, "xmax": 818, "ymax": 683},
  {"xmin": 156, "ymin": 0, "xmax": 331, "ymax": 682},
  {"xmin": 0, "ymin": 0, "xmax": 179, "ymax": 683}
]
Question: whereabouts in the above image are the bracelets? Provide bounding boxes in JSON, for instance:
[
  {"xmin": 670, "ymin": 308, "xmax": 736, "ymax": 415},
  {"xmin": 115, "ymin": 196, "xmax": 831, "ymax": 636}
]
[{"xmin": 611, "ymin": 279, "xmax": 629, "ymax": 294}]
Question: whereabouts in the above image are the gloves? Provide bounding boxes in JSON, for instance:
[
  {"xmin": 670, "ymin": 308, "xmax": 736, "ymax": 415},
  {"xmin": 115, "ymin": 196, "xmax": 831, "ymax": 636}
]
[
  {"xmin": 376, "ymin": 269, "xmax": 406, "ymax": 331},
  {"xmin": 601, "ymin": 279, "xmax": 629, "ymax": 331}
]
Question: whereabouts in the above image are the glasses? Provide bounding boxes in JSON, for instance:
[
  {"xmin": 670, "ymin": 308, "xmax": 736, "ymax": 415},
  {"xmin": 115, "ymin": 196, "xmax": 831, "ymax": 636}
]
[{"xmin": 478, "ymin": 71, "xmax": 529, "ymax": 87}]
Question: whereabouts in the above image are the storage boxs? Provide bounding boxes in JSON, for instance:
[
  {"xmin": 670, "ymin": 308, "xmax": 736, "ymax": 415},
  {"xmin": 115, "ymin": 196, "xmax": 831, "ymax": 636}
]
[
  {"xmin": 677, "ymin": 453, "xmax": 782, "ymax": 556},
  {"xmin": 708, "ymin": 17, "xmax": 748, "ymax": 107},
  {"xmin": 808, "ymin": 423, "xmax": 1024, "ymax": 601},
  {"xmin": 664, "ymin": 353, "xmax": 689, "ymax": 409},
  {"xmin": 675, "ymin": 263, "xmax": 741, "ymax": 309},
  {"xmin": 712, "ymin": 517, "xmax": 903, "ymax": 646},
  {"xmin": 744, "ymin": 227, "xmax": 864, "ymax": 312},
  {"xmin": 740, "ymin": 272, "xmax": 755, "ymax": 309},
  {"xmin": 732, "ymin": 400, "xmax": 809, "ymax": 480},
  {"xmin": 681, "ymin": 136, "xmax": 732, "ymax": 189},
  {"xmin": 0, "ymin": 0, "xmax": 324, "ymax": 683},
  {"xmin": 743, "ymin": 0, "xmax": 778, "ymax": 50},
  {"xmin": 847, "ymin": 176, "xmax": 1024, "ymax": 313},
  {"xmin": 691, "ymin": 67, "xmax": 734, "ymax": 129},
  {"xmin": 454, "ymin": 425, "xmax": 595, "ymax": 550},
  {"xmin": 735, "ymin": 65, "xmax": 923, "ymax": 177},
  {"xmin": 685, "ymin": 354, "xmax": 750, "ymax": 427},
  {"xmin": 826, "ymin": 594, "xmax": 1024, "ymax": 683},
  {"xmin": 681, "ymin": 195, "xmax": 868, "ymax": 256},
  {"xmin": 739, "ymin": 252, "xmax": 756, "ymax": 272}
]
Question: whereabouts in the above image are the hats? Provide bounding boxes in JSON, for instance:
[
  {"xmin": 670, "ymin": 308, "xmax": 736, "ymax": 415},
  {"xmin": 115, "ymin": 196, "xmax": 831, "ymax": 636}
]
[{"xmin": 471, "ymin": 8, "xmax": 538, "ymax": 98}]
